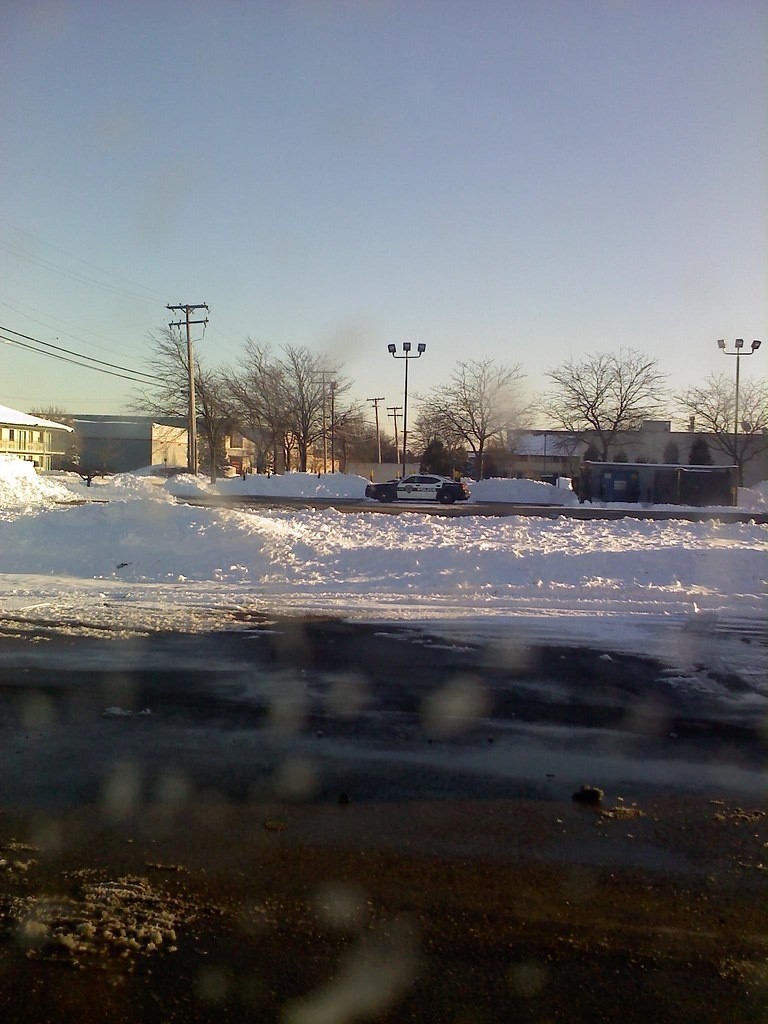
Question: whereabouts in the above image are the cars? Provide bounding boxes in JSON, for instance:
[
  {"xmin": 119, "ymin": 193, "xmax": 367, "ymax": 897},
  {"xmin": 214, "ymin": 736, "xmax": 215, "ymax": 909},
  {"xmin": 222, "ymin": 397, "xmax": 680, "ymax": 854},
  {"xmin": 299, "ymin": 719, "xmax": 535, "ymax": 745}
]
[{"xmin": 364, "ymin": 473, "xmax": 473, "ymax": 505}]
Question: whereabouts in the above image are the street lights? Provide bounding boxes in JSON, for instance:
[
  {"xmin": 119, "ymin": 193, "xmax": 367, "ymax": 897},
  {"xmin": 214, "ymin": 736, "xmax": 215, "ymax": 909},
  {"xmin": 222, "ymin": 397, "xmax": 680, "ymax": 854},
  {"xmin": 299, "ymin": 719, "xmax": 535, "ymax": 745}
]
[
  {"xmin": 388, "ymin": 342, "xmax": 427, "ymax": 479},
  {"xmin": 717, "ymin": 338, "xmax": 761, "ymax": 466}
]
